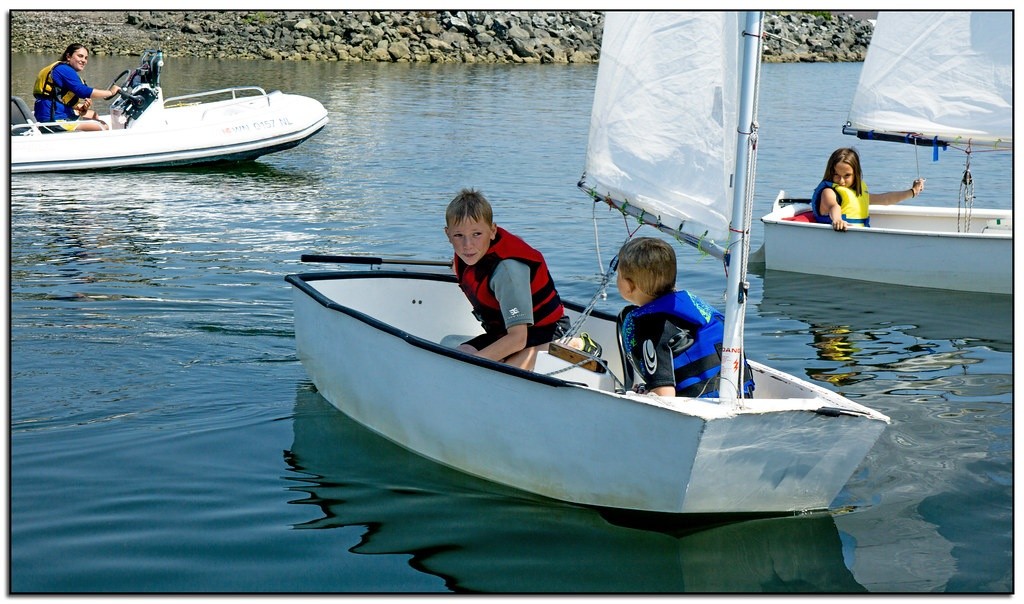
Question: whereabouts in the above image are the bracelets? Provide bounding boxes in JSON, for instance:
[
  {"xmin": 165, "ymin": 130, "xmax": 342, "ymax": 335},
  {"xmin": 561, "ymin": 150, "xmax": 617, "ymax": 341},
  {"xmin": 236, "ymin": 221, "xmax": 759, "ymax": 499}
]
[{"xmin": 910, "ymin": 189, "xmax": 914, "ymax": 197}]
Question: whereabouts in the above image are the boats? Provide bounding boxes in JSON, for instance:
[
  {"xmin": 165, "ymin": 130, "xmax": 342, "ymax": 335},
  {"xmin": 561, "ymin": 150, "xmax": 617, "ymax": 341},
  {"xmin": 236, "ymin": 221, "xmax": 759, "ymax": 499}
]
[{"xmin": 11, "ymin": 50, "xmax": 329, "ymax": 174}]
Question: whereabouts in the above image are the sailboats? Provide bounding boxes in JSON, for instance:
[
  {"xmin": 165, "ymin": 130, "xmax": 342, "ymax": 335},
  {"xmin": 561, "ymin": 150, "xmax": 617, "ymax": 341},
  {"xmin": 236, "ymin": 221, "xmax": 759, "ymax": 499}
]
[
  {"xmin": 759, "ymin": 11, "xmax": 1012, "ymax": 295},
  {"xmin": 284, "ymin": 11, "xmax": 891, "ymax": 514}
]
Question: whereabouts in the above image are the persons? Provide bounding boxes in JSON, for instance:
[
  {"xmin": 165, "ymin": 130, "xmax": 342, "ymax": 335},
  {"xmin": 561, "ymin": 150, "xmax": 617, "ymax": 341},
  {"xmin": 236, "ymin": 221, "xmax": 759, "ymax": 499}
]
[
  {"xmin": 443, "ymin": 192, "xmax": 570, "ymax": 372},
  {"xmin": 617, "ymin": 239, "xmax": 754, "ymax": 399},
  {"xmin": 34, "ymin": 44, "xmax": 121, "ymax": 133},
  {"xmin": 812, "ymin": 148, "xmax": 924, "ymax": 231}
]
